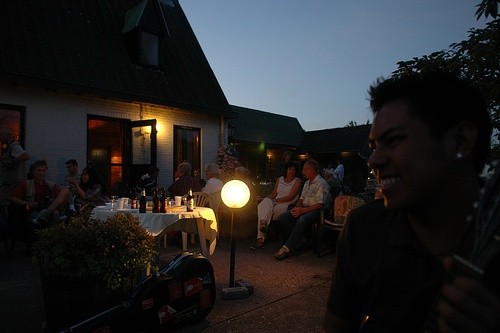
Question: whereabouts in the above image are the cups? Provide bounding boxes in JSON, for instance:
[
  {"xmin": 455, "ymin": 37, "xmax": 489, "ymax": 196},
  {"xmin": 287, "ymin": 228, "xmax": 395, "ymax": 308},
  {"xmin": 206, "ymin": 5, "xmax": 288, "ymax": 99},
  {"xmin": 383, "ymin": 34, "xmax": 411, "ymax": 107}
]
[
  {"xmin": 121, "ymin": 198, "xmax": 129, "ymax": 208},
  {"xmin": 111, "ymin": 196, "xmax": 118, "ymax": 208},
  {"xmin": 175, "ymin": 196, "xmax": 182, "ymax": 207}
]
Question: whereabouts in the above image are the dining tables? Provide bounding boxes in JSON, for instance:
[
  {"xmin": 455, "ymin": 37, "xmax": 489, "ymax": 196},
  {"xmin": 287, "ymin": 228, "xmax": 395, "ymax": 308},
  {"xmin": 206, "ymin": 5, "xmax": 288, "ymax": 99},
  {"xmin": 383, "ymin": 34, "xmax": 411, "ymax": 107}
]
[{"xmin": 91, "ymin": 205, "xmax": 218, "ymax": 277}]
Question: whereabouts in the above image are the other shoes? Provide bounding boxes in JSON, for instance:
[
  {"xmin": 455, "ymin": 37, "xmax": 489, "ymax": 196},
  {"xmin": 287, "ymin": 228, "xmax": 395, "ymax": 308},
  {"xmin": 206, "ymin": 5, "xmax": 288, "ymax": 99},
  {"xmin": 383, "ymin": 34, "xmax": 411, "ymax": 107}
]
[
  {"xmin": 58, "ymin": 211, "xmax": 67, "ymax": 220},
  {"xmin": 273, "ymin": 246, "xmax": 289, "ymax": 260},
  {"xmin": 249, "ymin": 238, "xmax": 265, "ymax": 250}
]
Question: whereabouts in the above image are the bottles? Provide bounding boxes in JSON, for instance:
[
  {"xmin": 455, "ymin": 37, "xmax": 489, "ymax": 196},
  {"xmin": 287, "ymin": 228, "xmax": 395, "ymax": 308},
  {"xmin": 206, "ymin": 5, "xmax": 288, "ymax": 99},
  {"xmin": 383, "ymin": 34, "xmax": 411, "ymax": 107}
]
[
  {"xmin": 367, "ymin": 170, "xmax": 376, "ymax": 190},
  {"xmin": 131, "ymin": 194, "xmax": 137, "ymax": 209},
  {"xmin": 165, "ymin": 197, "xmax": 174, "ymax": 208},
  {"xmin": 138, "ymin": 188, "xmax": 146, "ymax": 213},
  {"xmin": 159, "ymin": 187, "xmax": 168, "ymax": 213},
  {"xmin": 182, "ymin": 188, "xmax": 193, "ymax": 212},
  {"xmin": 151, "ymin": 187, "xmax": 159, "ymax": 213}
]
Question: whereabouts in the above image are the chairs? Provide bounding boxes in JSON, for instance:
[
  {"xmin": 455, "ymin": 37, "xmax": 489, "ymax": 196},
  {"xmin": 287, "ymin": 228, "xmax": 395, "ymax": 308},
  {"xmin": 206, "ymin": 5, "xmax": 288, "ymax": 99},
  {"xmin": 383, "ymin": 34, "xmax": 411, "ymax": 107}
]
[
  {"xmin": 181, "ymin": 192, "xmax": 212, "ymax": 250},
  {"xmin": 321, "ymin": 193, "xmax": 370, "ymax": 257}
]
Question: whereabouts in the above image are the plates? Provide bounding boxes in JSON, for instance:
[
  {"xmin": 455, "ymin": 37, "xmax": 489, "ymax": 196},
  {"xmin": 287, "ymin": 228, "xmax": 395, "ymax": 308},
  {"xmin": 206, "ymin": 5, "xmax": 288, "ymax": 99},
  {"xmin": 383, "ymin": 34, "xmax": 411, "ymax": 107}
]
[
  {"xmin": 146, "ymin": 206, "xmax": 153, "ymax": 210},
  {"xmin": 116, "ymin": 209, "xmax": 139, "ymax": 212},
  {"xmin": 97, "ymin": 206, "xmax": 111, "ymax": 209}
]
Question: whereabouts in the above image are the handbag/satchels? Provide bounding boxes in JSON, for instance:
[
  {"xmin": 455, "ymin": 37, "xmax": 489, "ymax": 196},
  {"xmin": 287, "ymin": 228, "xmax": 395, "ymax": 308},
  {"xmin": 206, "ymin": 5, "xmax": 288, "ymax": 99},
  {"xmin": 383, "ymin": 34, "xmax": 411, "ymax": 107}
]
[{"xmin": 334, "ymin": 194, "xmax": 368, "ymax": 223}]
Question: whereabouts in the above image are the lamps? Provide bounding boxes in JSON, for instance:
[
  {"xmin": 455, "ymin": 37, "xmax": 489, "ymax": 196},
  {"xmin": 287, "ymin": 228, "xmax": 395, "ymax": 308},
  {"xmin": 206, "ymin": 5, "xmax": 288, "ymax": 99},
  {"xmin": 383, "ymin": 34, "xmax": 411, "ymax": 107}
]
[{"xmin": 220, "ymin": 180, "xmax": 250, "ymax": 289}]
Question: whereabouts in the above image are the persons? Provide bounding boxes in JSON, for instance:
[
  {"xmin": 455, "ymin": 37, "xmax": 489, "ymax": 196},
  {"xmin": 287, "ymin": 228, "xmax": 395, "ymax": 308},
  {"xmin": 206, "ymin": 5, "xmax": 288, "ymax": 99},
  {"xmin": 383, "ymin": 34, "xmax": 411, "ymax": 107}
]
[
  {"xmin": 194, "ymin": 170, "xmax": 207, "ymax": 188},
  {"xmin": 59, "ymin": 159, "xmax": 83, "ymax": 205},
  {"xmin": 175, "ymin": 171, "xmax": 180, "ymax": 181},
  {"xmin": 330, "ymin": 157, "xmax": 344, "ymax": 196},
  {"xmin": 235, "ymin": 167, "xmax": 249, "ymax": 181},
  {"xmin": 250, "ymin": 161, "xmax": 301, "ymax": 250},
  {"xmin": 6, "ymin": 160, "xmax": 69, "ymax": 225},
  {"xmin": 201, "ymin": 163, "xmax": 224, "ymax": 207},
  {"xmin": 318, "ymin": 72, "xmax": 500, "ymax": 333},
  {"xmin": 168, "ymin": 162, "xmax": 203, "ymax": 198},
  {"xmin": 65, "ymin": 167, "xmax": 107, "ymax": 215},
  {"xmin": 273, "ymin": 160, "xmax": 330, "ymax": 260}
]
